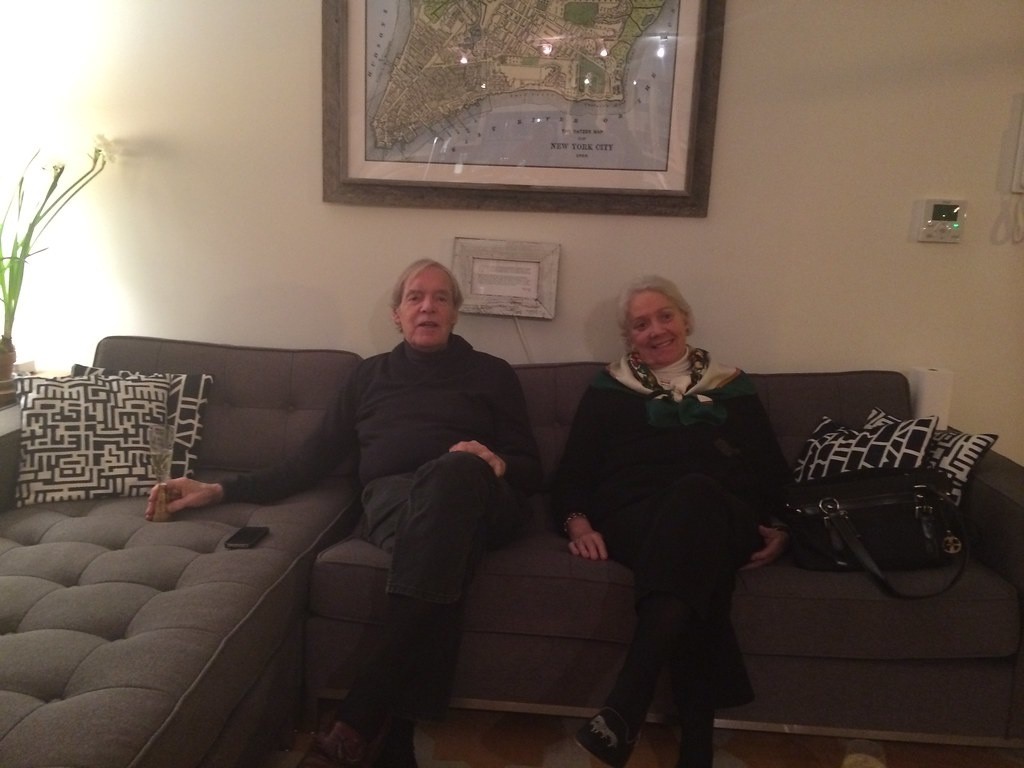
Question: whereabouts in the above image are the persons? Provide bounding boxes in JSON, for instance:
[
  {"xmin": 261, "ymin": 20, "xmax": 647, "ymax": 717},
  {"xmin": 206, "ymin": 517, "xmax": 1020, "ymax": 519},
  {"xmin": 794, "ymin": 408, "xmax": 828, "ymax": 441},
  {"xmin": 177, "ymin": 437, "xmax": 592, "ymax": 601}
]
[
  {"xmin": 540, "ymin": 276, "xmax": 796, "ymax": 768},
  {"xmin": 143, "ymin": 257, "xmax": 547, "ymax": 768}
]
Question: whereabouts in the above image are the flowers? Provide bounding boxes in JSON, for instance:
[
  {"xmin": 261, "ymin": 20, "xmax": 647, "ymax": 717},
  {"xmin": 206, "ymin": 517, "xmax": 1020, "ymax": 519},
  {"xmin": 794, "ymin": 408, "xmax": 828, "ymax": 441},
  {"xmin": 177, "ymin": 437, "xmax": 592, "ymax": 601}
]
[{"xmin": 1, "ymin": 132, "xmax": 122, "ymax": 356}]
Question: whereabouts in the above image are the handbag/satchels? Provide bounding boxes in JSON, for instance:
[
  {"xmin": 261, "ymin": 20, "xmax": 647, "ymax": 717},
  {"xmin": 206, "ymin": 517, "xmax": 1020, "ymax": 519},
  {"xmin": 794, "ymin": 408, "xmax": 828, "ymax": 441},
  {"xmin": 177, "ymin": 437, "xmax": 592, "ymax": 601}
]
[{"xmin": 782, "ymin": 467, "xmax": 966, "ymax": 599}]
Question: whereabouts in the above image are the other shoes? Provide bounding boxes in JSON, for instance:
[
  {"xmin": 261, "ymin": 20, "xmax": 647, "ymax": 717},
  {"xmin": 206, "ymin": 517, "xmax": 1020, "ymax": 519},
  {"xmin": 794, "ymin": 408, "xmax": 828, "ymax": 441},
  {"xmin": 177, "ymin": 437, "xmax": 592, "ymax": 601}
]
[
  {"xmin": 297, "ymin": 709, "xmax": 392, "ymax": 768},
  {"xmin": 574, "ymin": 696, "xmax": 648, "ymax": 768}
]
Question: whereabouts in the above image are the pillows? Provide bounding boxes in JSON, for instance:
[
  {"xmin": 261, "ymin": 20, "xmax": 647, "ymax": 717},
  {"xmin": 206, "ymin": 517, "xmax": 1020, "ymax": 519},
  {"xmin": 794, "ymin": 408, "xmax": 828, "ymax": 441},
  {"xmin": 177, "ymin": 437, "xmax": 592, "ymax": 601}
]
[
  {"xmin": 793, "ymin": 415, "xmax": 939, "ymax": 483},
  {"xmin": 72, "ymin": 363, "xmax": 214, "ymax": 478},
  {"xmin": 861, "ymin": 406, "xmax": 999, "ymax": 510},
  {"xmin": 13, "ymin": 372, "xmax": 171, "ymax": 509}
]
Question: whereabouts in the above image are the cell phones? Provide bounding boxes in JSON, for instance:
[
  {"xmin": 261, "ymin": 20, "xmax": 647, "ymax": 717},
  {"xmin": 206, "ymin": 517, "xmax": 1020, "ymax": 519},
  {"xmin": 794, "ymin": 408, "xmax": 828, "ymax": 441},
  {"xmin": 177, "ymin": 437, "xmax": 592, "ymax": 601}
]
[{"xmin": 225, "ymin": 526, "xmax": 270, "ymax": 548}]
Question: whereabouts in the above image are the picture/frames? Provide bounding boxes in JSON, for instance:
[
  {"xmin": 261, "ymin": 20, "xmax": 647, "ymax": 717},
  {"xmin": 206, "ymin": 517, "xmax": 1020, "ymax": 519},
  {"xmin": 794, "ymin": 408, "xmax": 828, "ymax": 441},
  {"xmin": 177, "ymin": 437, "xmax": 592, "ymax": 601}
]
[
  {"xmin": 451, "ymin": 236, "xmax": 561, "ymax": 322},
  {"xmin": 320, "ymin": 0, "xmax": 726, "ymax": 219}
]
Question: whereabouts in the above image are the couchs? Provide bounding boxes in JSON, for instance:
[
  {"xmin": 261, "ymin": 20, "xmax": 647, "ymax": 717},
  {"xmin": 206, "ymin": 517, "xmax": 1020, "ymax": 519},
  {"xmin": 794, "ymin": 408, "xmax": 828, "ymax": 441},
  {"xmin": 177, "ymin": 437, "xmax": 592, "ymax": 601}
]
[
  {"xmin": 0, "ymin": 334, "xmax": 364, "ymax": 768},
  {"xmin": 299, "ymin": 363, "xmax": 1024, "ymax": 738}
]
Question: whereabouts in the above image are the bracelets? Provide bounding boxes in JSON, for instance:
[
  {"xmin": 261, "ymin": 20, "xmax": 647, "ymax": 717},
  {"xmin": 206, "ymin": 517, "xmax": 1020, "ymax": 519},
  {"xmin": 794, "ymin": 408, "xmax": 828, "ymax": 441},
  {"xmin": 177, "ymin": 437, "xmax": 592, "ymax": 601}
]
[{"xmin": 562, "ymin": 510, "xmax": 588, "ymax": 536}]
